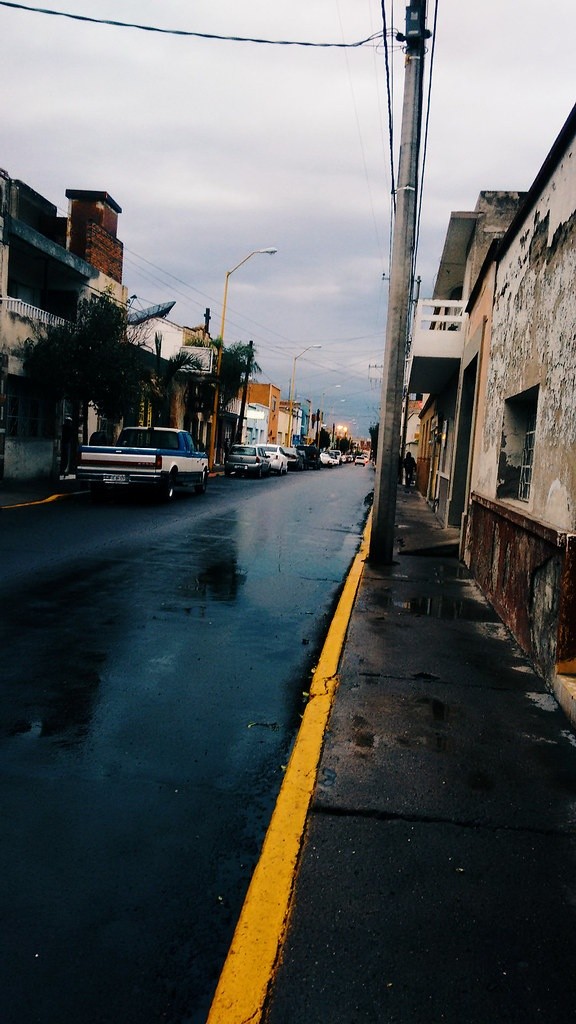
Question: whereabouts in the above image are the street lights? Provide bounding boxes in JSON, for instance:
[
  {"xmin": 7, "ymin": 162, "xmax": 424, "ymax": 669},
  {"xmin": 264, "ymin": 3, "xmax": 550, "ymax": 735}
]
[
  {"xmin": 285, "ymin": 343, "xmax": 322, "ymax": 449},
  {"xmin": 317, "ymin": 383, "xmax": 343, "ymax": 447},
  {"xmin": 210, "ymin": 247, "xmax": 278, "ymax": 489},
  {"xmin": 328, "ymin": 399, "xmax": 346, "ymax": 446}
]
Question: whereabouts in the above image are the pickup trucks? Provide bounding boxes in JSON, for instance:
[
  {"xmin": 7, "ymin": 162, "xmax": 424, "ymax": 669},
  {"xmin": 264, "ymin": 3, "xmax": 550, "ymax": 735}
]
[{"xmin": 77, "ymin": 428, "xmax": 212, "ymax": 502}]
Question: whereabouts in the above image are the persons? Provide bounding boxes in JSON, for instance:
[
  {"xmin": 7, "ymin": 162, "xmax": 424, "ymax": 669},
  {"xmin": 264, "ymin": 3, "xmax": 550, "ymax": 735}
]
[{"xmin": 402, "ymin": 452, "xmax": 416, "ymax": 487}]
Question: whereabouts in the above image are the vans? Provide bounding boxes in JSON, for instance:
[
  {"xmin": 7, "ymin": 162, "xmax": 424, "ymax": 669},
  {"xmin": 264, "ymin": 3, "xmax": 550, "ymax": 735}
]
[
  {"xmin": 355, "ymin": 456, "xmax": 366, "ymax": 467},
  {"xmin": 299, "ymin": 444, "xmax": 322, "ymax": 470}
]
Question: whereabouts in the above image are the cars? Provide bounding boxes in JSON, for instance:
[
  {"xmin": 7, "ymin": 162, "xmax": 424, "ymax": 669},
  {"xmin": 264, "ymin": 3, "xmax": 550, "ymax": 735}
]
[
  {"xmin": 320, "ymin": 448, "xmax": 354, "ymax": 467},
  {"xmin": 226, "ymin": 444, "xmax": 272, "ymax": 477},
  {"xmin": 284, "ymin": 447, "xmax": 304, "ymax": 470},
  {"xmin": 258, "ymin": 445, "xmax": 289, "ymax": 474}
]
